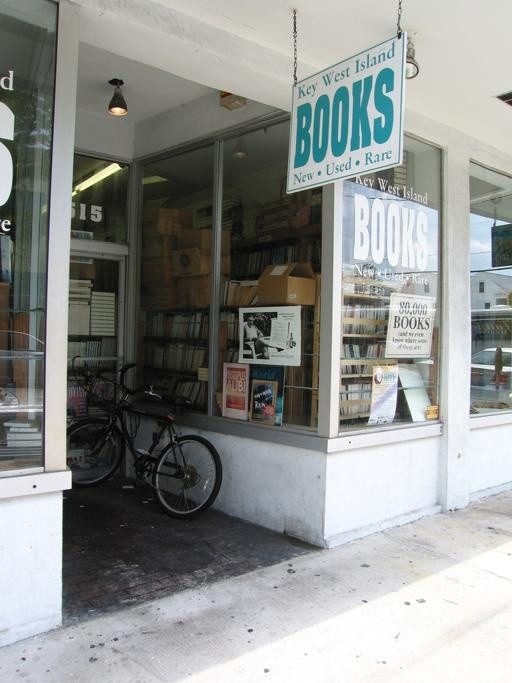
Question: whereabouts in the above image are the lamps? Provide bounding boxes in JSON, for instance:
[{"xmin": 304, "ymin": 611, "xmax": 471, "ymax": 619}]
[
  {"xmin": 405, "ymin": 28, "xmax": 422, "ymax": 80},
  {"xmin": 107, "ymin": 78, "xmax": 129, "ymax": 116}
]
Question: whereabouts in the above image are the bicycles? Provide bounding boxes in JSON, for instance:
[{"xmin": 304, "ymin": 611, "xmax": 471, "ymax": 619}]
[{"xmin": 66, "ymin": 359, "xmax": 222, "ymax": 521}]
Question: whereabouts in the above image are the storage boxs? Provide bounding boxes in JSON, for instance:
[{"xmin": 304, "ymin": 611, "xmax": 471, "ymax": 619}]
[{"xmin": 140, "ymin": 206, "xmax": 318, "ymax": 309}]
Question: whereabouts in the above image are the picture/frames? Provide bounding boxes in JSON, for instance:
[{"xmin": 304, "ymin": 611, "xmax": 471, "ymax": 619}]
[{"xmin": 249, "ymin": 379, "xmax": 279, "ymax": 424}]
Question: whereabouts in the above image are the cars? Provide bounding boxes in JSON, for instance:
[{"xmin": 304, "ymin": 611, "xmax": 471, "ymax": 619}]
[{"xmin": 470, "ymin": 347, "xmax": 512, "ymax": 402}]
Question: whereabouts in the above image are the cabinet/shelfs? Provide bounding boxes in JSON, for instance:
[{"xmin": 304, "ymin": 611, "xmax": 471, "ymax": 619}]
[
  {"xmin": 139, "ymin": 334, "xmax": 209, "ymax": 414},
  {"xmin": 309, "ymin": 271, "xmax": 403, "ymax": 426}
]
